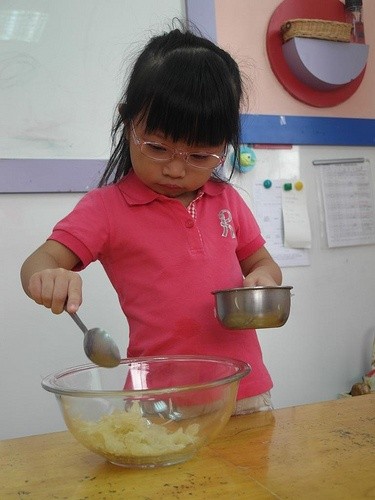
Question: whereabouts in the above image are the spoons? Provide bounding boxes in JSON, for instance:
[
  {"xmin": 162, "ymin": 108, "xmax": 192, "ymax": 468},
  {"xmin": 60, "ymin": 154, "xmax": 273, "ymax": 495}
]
[{"xmin": 64, "ymin": 304, "xmax": 121, "ymax": 368}]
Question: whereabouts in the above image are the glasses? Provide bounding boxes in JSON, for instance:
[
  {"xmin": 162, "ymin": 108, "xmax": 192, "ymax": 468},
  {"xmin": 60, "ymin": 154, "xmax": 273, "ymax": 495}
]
[{"xmin": 128, "ymin": 117, "xmax": 227, "ymax": 170}]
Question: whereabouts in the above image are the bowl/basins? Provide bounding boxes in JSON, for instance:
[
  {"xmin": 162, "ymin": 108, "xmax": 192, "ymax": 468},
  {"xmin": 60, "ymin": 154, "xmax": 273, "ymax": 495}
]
[
  {"xmin": 40, "ymin": 355, "xmax": 251, "ymax": 467},
  {"xmin": 211, "ymin": 286, "xmax": 293, "ymax": 329}
]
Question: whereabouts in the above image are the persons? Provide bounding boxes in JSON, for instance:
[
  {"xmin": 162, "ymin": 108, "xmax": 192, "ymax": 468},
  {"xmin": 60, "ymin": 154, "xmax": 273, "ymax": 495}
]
[{"xmin": 20, "ymin": 33, "xmax": 282, "ymax": 414}]
